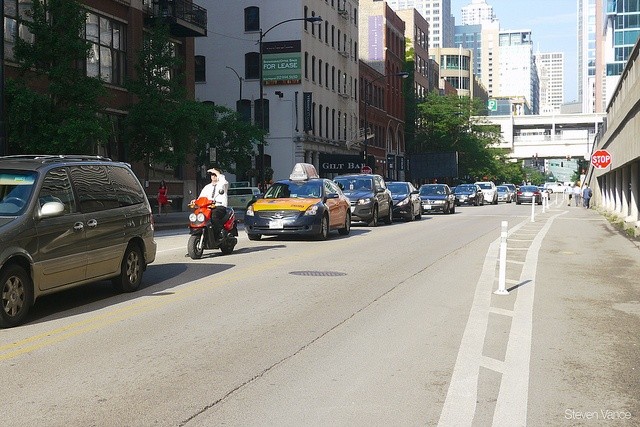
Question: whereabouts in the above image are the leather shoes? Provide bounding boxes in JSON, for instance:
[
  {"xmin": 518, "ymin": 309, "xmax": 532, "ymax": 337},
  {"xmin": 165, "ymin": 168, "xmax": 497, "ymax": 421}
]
[
  {"xmin": 185, "ymin": 253, "xmax": 190, "ymax": 257},
  {"xmin": 220, "ymin": 228, "xmax": 226, "ymax": 236}
]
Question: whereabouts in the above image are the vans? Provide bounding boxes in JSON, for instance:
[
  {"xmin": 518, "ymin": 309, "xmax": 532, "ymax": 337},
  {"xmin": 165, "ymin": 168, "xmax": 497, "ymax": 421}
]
[
  {"xmin": 0, "ymin": 155, "xmax": 156, "ymax": 328},
  {"xmin": 504, "ymin": 184, "xmax": 516, "ymax": 202},
  {"xmin": 228, "ymin": 187, "xmax": 259, "ymax": 210},
  {"xmin": 333, "ymin": 173, "xmax": 393, "ymax": 226},
  {"xmin": 544, "ymin": 182, "xmax": 566, "ymax": 193},
  {"xmin": 474, "ymin": 181, "xmax": 498, "ymax": 205}
]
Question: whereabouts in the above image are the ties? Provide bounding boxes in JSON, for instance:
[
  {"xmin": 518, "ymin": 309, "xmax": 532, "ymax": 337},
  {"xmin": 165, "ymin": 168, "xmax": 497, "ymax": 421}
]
[{"xmin": 212, "ymin": 184, "xmax": 216, "ymax": 198}]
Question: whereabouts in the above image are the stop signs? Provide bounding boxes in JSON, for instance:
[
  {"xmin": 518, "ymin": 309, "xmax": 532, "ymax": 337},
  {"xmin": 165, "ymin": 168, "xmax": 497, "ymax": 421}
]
[
  {"xmin": 361, "ymin": 166, "xmax": 372, "ymax": 175},
  {"xmin": 591, "ymin": 150, "xmax": 612, "ymax": 170}
]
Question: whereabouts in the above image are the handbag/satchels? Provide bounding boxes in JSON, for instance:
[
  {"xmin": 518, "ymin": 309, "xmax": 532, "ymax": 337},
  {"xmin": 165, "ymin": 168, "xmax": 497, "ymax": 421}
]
[{"xmin": 588, "ymin": 191, "xmax": 592, "ymax": 197}]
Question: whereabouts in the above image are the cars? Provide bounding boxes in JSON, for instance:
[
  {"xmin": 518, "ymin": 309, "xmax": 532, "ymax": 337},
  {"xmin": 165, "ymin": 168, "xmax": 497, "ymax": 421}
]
[
  {"xmin": 450, "ymin": 187, "xmax": 456, "ymax": 193},
  {"xmin": 418, "ymin": 184, "xmax": 455, "ymax": 214},
  {"xmin": 538, "ymin": 187, "xmax": 550, "ymax": 200},
  {"xmin": 495, "ymin": 186, "xmax": 511, "ymax": 202},
  {"xmin": 453, "ymin": 184, "xmax": 484, "ymax": 206},
  {"xmin": 246, "ymin": 163, "xmax": 351, "ymax": 240},
  {"xmin": 517, "ymin": 186, "xmax": 542, "ymax": 205},
  {"xmin": 548, "ymin": 189, "xmax": 553, "ymax": 193},
  {"xmin": 385, "ymin": 182, "xmax": 421, "ymax": 220}
]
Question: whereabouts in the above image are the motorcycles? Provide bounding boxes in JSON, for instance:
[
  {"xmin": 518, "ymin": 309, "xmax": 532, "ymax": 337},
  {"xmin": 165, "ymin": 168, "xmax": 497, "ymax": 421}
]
[{"xmin": 187, "ymin": 197, "xmax": 238, "ymax": 259}]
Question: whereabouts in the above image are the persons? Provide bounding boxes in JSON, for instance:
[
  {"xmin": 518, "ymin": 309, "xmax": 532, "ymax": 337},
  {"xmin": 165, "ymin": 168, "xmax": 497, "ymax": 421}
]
[
  {"xmin": 257, "ymin": 178, "xmax": 273, "ymax": 194},
  {"xmin": 191, "ymin": 168, "xmax": 229, "ymax": 239},
  {"xmin": 582, "ymin": 183, "xmax": 590, "ymax": 197},
  {"xmin": 583, "ymin": 186, "xmax": 592, "ymax": 208},
  {"xmin": 156, "ymin": 180, "xmax": 169, "ymax": 216},
  {"xmin": 573, "ymin": 185, "xmax": 582, "ymax": 206},
  {"xmin": 566, "ymin": 184, "xmax": 573, "ymax": 205}
]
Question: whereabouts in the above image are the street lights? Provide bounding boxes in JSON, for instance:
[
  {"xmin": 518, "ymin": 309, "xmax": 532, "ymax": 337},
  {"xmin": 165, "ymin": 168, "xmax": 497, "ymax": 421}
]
[
  {"xmin": 364, "ymin": 72, "xmax": 410, "ymax": 165},
  {"xmin": 225, "ymin": 65, "xmax": 242, "ymax": 116},
  {"xmin": 259, "ymin": 16, "xmax": 323, "ymax": 193}
]
